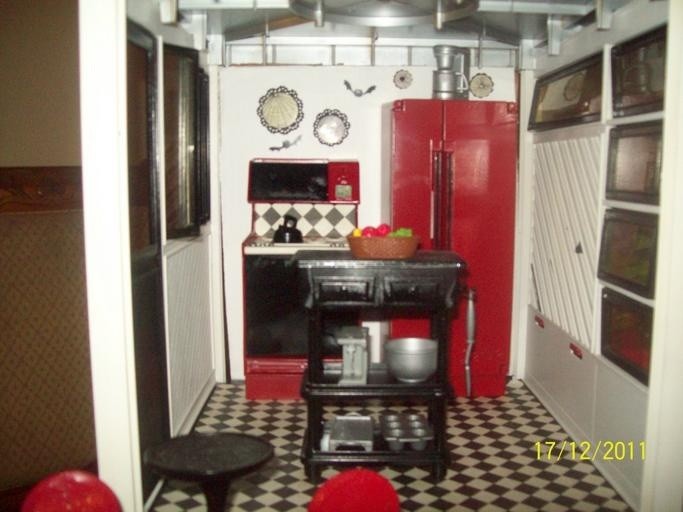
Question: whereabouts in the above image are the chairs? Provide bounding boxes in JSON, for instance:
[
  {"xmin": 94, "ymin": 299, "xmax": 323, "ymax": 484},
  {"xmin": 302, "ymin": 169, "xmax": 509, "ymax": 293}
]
[
  {"xmin": 306, "ymin": 473, "xmax": 399, "ymax": 510},
  {"xmin": 18, "ymin": 473, "xmax": 124, "ymax": 509}
]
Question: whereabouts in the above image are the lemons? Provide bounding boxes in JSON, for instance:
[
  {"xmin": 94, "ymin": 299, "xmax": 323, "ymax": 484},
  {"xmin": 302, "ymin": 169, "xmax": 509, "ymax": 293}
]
[{"xmin": 352, "ymin": 228, "xmax": 362, "ymax": 237}]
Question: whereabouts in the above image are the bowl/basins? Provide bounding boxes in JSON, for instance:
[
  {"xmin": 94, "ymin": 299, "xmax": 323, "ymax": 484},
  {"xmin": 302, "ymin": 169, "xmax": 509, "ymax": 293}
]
[{"xmin": 384, "ymin": 337, "xmax": 440, "ymax": 384}]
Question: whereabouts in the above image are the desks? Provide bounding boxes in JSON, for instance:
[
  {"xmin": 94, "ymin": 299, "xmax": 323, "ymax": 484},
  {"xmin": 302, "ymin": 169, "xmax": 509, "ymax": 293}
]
[{"xmin": 286, "ymin": 249, "xmax": 466, "ymax": 485}]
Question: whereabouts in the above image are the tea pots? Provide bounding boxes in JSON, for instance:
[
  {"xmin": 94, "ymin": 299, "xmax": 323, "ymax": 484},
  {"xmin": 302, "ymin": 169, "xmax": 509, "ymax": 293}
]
[{"xmin": 273, "ymin": 214, "xmax": 302, "ymax": 243}]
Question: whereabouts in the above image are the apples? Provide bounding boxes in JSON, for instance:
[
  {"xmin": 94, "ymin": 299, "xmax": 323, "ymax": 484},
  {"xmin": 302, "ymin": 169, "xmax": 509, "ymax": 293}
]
[{"xmin": 362, "ymin": 223, "xmax": 412, "ymax": 238}]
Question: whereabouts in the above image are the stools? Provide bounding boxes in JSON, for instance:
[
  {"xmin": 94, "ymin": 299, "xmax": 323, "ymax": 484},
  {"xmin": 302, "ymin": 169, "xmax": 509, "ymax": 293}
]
[{"xmin": 140, "ymin": 432, "xmax": 275, "ymax": 512}]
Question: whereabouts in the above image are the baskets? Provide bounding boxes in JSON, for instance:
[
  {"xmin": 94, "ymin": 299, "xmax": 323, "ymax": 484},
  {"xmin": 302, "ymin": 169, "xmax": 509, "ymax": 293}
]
[{"xmin": 347, "ymin": 234, "xmax": 421, "ymax": 260}]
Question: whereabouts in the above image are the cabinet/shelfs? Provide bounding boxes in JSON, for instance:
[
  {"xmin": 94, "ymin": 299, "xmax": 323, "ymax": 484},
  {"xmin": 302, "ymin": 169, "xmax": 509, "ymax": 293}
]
[{"xmin": 525, "ymin": 0, "xmax": 683, "ymax": 512}]
